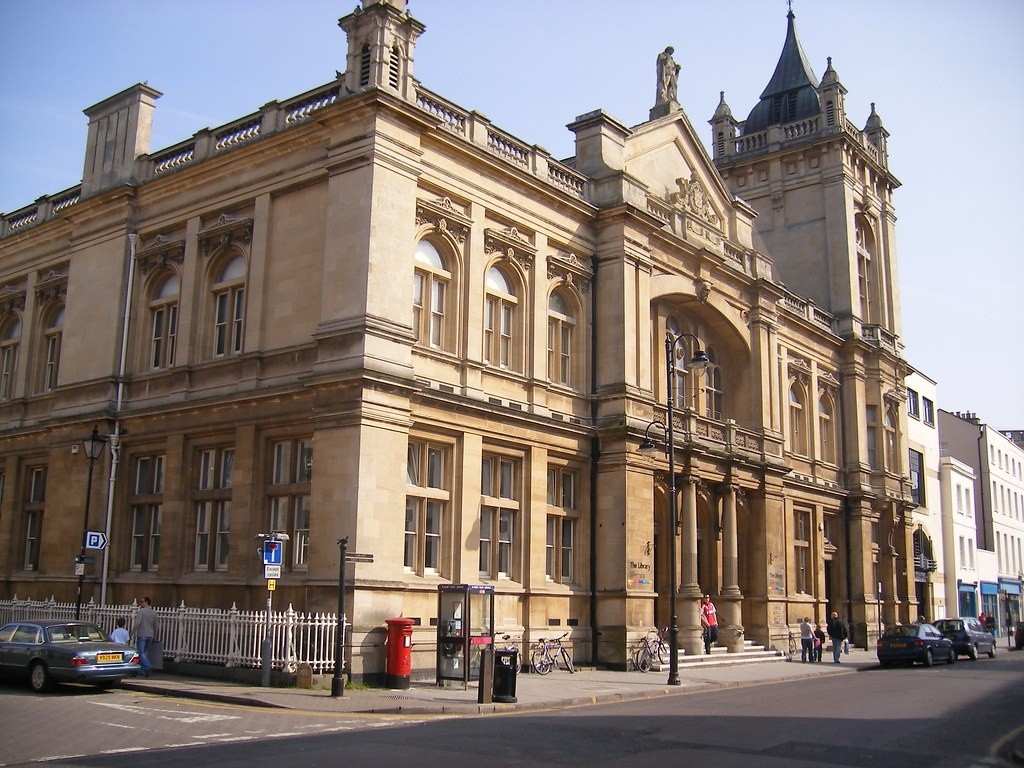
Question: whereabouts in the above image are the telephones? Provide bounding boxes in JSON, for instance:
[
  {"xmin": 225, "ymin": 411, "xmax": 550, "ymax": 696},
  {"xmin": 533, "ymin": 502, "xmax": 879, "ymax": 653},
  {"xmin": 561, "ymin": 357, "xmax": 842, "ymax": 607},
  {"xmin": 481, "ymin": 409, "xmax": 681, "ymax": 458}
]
[{"xmin": 446, "ymin": 620, "xmax": 456, "ymax": 637}]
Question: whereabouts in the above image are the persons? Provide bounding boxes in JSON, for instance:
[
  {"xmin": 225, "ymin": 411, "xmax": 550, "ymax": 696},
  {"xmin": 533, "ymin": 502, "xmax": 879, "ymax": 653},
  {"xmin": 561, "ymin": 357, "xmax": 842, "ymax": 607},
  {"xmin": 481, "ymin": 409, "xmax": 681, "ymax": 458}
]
[
  {"xmin": 800, "ymin": 617, "xmax": 819, "ymax": 663},
  {"xmin": 915, "ymin": 616, "xmax": 925, "ymax": 624},
  {"xmin": 110, "ymin": 617, "xmax": 130, "ymax": 645},
  {"xmin": 700, "ymin": 594, "xmax": 718, "ymax": 654},
  {"xmin": 827, "ymin": 612, "xmax": 849, "ymax": 663},
  {"xmin": 979, "ymin": 612, "xmax": 997, "ymax": 638},
  {"xmin": 812, "ymin": 624, "xmax": 825, "ymax": 662},
  {"xmin": 129, "ymin": 597, "xmax": 159, "ymax": 677},
  {"xmin": 657, "ymin": 46, "xmax": 681, "ymax": 99},
  {"xmin": 698, "ymin": 282, "xmax": 712, "ymax": 302}
]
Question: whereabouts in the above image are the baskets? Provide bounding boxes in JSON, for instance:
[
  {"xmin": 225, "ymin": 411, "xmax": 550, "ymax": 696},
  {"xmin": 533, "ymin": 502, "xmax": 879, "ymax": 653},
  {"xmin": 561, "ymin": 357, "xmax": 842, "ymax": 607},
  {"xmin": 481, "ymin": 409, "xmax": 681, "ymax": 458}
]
[{"xmin": 481, "ymin": 633, "xmax": 491, "ymax": 637}]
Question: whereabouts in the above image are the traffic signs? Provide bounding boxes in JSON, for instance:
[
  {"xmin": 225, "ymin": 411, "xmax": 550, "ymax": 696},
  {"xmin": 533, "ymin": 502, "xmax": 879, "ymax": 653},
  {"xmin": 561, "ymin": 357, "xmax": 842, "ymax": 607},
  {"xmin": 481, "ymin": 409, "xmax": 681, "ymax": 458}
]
[{"xmin": 345, "ymin": 552, "xmax": 374, "ymax": 563}]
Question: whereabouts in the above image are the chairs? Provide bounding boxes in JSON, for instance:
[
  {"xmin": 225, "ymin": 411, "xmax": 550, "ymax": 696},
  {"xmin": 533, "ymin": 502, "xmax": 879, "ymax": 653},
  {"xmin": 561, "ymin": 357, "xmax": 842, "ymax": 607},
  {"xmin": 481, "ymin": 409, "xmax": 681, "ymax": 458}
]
[
  {"xmin": 51, "ymin": 633, "xmax": 63, "ymax": 640},
  {"xmin": 88, "ymin": 632, "xmax": 99, "ymax": 640}
]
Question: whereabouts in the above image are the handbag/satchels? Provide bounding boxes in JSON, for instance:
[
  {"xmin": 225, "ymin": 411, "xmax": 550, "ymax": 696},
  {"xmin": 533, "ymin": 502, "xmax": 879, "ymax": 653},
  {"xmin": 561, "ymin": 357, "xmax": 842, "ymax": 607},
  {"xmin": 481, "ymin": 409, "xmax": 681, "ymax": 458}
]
[{"xmin": 844, "ymin": 639, "xmax": 848, "ymax": 654}]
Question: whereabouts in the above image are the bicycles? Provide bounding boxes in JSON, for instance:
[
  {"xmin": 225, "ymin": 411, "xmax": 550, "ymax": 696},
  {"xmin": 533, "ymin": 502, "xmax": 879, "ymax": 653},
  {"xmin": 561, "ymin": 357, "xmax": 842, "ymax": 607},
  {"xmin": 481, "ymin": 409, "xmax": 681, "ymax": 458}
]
[
  {"xmin": 532, "ymin": 632, "xmax": 574, "ymax": 675},
  {"xmin": 470, "ymin": 630, "xmax": 522, "ymax": 676},
  {"xmin": 788, "ymin": 631, "xmax": 797, "ymax": 652},
  {"xmin": 635, "ymin": 627, "xmax": 671, "ymax": 672}
]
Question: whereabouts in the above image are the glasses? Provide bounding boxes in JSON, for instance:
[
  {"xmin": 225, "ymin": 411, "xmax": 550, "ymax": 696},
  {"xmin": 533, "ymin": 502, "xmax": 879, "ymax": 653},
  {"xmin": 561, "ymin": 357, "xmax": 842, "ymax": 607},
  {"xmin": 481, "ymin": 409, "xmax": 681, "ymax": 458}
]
[{"xmin": 139, "ymin": 601, "xmax": 145, "ymax": 603}]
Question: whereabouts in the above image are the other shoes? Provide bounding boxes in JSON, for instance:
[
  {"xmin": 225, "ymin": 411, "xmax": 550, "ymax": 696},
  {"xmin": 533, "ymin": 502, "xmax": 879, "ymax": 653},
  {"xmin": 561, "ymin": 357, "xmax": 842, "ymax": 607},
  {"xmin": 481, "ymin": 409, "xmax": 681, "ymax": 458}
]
[{"xmin": 145, "ymin": 668, "xmax": 153, "ymax": 678}]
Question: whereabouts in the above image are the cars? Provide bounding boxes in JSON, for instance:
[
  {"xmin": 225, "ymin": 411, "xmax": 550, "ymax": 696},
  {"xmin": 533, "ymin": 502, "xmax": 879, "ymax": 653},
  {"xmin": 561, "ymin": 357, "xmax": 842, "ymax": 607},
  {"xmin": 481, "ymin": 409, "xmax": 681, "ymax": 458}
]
[
  {"xmin": 0, "ymin": 618, "xmax": 142, "ymax": 693},
  {"xmin": 876, "ymin": 623, "xmax": 957, "ymax": 668},
  {"xmin": 932, "ymin": 617, "xmax": 997, "ymax": 660}
]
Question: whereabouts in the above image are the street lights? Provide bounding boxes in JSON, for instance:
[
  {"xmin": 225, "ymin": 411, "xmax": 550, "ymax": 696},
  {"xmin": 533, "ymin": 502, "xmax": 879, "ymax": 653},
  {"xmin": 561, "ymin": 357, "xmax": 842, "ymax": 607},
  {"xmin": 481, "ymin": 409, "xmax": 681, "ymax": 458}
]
[
  {"xmin": 73, "ymin": 424, "xmax": 108, "ymax": 619},
  {"xmin": 635, "ymin": 333, "xmax": 715, "ymax": 687}
]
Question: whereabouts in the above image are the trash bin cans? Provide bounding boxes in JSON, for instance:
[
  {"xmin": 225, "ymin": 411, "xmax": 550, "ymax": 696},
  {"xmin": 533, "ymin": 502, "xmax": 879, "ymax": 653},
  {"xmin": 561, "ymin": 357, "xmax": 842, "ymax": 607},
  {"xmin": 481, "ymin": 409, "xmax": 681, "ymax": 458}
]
[{"xmin": 493, "ymin": 648, "xmax": 518, "ymax": 703}]
